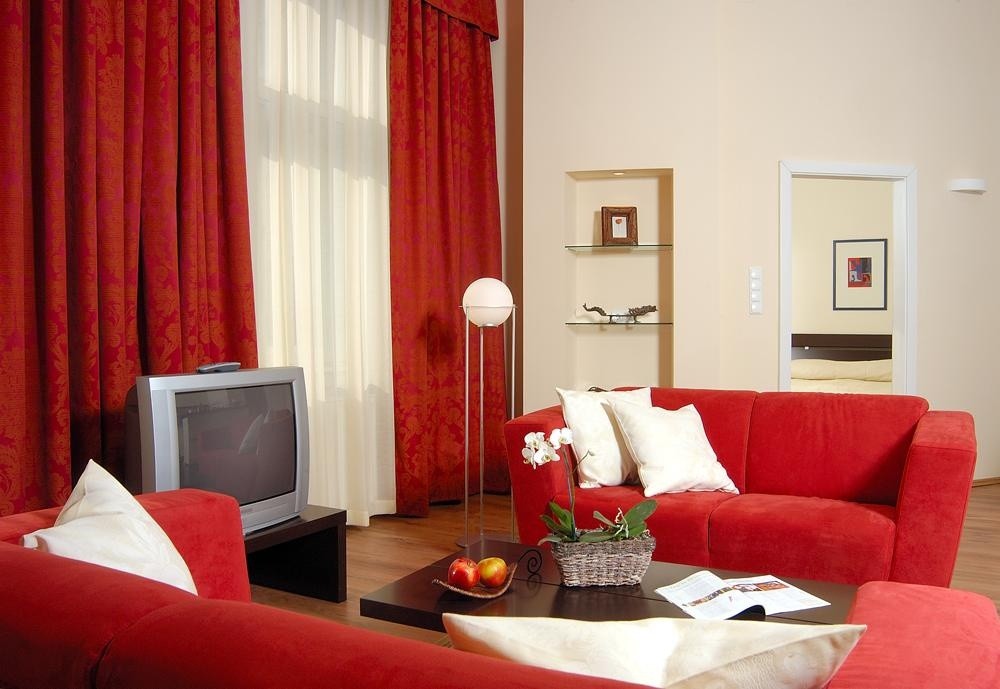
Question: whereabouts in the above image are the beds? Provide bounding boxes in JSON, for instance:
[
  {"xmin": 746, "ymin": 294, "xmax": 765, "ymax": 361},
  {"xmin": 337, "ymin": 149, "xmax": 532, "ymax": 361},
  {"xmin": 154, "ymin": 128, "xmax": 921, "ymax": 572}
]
[{"xmin": 790, "ymin": 334, "xmax": 892, "ymax": 395}]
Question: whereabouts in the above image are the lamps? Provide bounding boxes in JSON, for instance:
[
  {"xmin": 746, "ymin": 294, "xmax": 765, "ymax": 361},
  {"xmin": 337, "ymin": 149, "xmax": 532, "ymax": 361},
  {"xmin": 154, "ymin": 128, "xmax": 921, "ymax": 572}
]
[{"xmin": 459, "ymin": 277, "xmax": 517, "ymax": 547}]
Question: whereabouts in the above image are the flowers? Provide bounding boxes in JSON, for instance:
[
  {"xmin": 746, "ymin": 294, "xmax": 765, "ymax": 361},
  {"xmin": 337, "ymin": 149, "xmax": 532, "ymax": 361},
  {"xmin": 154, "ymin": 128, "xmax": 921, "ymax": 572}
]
[{"xmin": 536, "ymin": 501, "xmax": 659, "ymax": 546}]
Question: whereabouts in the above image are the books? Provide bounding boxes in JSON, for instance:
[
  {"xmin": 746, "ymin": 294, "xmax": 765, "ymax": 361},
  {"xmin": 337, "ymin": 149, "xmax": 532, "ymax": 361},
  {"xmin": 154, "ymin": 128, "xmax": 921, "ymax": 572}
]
[{"xmin": 654, "ymin": 569, "xmax": 831, "ymax": 621}]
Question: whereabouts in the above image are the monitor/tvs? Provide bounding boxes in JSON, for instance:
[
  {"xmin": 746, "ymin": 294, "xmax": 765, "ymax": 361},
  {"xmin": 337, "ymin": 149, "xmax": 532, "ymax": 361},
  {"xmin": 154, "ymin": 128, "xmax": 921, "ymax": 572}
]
[{"xmin": 119, "ymin": 365, "xmax": 310, "ymax": 535}]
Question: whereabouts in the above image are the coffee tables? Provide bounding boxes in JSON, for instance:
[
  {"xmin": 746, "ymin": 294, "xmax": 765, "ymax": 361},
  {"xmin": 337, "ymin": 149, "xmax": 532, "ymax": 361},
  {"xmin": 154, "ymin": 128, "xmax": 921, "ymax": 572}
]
[{"xmin": 360, "ymin": 536, "xmax": 857, "ymax": 637}]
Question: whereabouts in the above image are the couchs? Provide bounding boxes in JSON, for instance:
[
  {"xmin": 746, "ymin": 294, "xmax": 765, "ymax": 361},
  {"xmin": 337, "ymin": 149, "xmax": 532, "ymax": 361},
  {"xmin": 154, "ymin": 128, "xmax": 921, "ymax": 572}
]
[
  {"xmin": 503, "ymin": 386, "xmax": 977, "ymax": 622},
  {"xmin": 0, "ymin": 486, "xmax": 1000, "ymax": 689}
]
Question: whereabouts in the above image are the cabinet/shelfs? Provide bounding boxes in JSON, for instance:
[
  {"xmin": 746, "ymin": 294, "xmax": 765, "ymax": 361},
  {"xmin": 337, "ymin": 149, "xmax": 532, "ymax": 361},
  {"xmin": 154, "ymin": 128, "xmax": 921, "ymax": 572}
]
[{"xmin": 566, "ymin": 171, "xmax": 674, "ymax": 389}]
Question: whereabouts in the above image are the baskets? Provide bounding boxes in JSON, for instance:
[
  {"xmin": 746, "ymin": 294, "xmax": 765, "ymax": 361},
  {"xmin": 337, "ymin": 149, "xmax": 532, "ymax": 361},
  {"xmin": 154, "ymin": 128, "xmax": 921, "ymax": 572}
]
[{"xmin": 549, "ymin": 528, "xmax": 657, "ymax": 588}]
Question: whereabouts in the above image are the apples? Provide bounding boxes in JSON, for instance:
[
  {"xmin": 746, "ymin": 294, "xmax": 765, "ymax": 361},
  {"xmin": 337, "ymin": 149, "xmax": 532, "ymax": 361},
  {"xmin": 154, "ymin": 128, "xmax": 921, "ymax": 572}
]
[
  {"xmin": 447, "ymin": 557, "xmax": 480, "ymax": 591},
  {"xmin": 477, "ymin": 557, "xmax": 508, "ymax": 588}
]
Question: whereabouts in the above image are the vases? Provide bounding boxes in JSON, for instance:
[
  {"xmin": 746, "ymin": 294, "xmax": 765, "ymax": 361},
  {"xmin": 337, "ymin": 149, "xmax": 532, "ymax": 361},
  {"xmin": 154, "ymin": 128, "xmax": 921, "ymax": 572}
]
[{"xmin": 550, "ymin": 529, "xmax": 656, "ymax": 587}]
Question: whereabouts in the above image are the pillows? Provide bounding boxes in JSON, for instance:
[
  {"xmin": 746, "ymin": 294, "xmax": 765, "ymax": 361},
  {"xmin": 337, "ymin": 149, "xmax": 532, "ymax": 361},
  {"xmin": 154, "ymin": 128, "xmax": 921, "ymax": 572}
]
[
  {"xmin": 791, "ymin": 358, "xmax": 893, "ymax": 384},
  {"xmin": 18, "ymin": 458, "xmax": 198, "ymax": 597},
  {"xmin": 441, "ymin": 612, "xmax": 867, "ymax": 689},
  {"xmin": 556, "ymin": 389, "xmax": 653, "ymax": 490},
  {"xmin": 604, "ymin": 396, "xmax": 739, "ymax": 500}
]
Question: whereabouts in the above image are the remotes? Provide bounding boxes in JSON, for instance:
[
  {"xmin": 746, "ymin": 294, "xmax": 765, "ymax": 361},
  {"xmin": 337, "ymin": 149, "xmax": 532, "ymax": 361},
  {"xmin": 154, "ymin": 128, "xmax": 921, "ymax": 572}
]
[{"xmin": 197, "ymin": 362, "xmax": 242, "ymax": 373}]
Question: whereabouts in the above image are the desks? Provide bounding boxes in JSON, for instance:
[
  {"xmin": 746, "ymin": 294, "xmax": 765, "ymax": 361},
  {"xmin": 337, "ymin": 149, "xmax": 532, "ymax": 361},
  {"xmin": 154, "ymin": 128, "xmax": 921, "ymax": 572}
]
[{"xmin": 237, "ymin": 504, "xmax": 350, "ymax": 605}]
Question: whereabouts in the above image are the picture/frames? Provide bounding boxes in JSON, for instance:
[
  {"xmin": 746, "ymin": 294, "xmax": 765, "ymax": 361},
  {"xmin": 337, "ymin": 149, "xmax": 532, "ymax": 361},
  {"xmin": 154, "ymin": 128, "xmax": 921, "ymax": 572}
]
[
  {"xmin": 602, "ymin": 206, "xmax": 638, "ymax": 245},
  {"xmin": 831, "ymin": 239, "xmax": 888, "ymax": 311}
]
